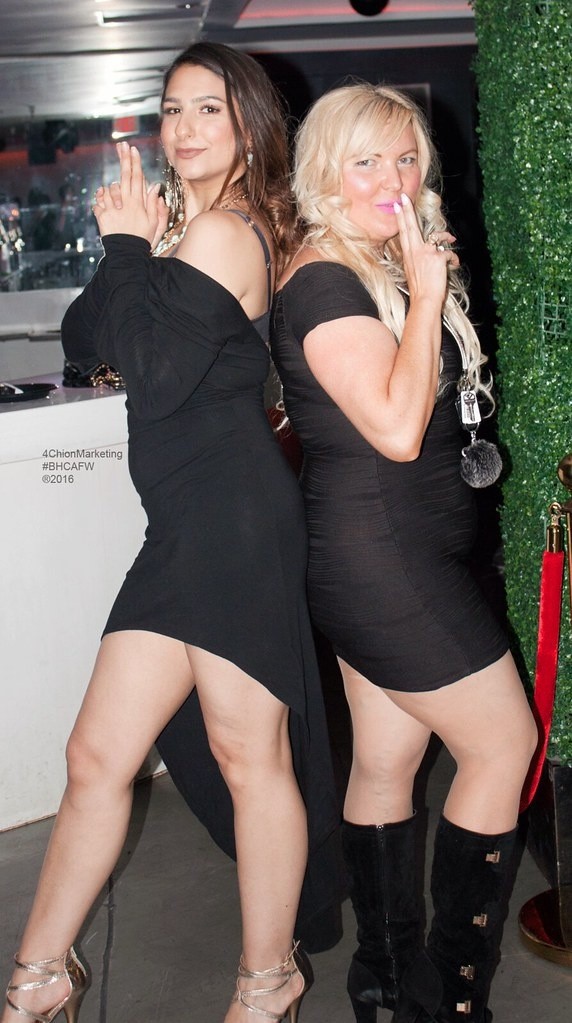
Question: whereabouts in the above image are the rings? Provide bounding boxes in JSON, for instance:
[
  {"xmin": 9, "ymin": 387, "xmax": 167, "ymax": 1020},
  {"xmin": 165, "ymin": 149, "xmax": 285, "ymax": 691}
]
[{"xmin": 428, "ymin": 233, "xmax": 438, "ymax": 245}]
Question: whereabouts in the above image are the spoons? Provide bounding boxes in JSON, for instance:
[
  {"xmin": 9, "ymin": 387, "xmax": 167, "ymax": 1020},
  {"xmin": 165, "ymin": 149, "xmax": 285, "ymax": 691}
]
[{"xmin": 3, "ymin": 382, "xmax": 24, "ymax": 394}]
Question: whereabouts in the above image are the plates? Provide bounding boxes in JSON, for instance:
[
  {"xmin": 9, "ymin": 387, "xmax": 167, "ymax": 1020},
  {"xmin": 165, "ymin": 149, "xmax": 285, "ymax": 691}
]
[{"xmin": 0, "ymin": 383, "xmax": 58, "ymax": 402}]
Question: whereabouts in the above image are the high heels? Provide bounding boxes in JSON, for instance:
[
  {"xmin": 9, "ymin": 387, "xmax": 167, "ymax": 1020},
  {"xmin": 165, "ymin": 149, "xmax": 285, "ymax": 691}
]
[
  {"xmin": 0, "ymin": 946, "xmax": 88, "ymax": 1023},
  {"xmin": 226, "ymin": 940, "xmax": 306, "ymax": 1023}
]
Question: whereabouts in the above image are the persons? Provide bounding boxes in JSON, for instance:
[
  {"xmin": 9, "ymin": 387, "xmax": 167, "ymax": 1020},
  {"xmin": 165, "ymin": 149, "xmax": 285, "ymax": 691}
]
[
  {"xmin": 5, "ymin": 40, "xmax": 309, "ymax": 1023},
  {"xmin": 269, "ymin": 83, "xmax": 538, "ymax": 1022}
]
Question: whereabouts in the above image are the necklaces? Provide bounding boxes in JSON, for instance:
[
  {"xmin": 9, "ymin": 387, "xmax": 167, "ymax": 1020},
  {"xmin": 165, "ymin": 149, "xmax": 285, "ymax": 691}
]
[{"xmin": 147, "ymin": 190, "xmax": 251, "ymax": 258}]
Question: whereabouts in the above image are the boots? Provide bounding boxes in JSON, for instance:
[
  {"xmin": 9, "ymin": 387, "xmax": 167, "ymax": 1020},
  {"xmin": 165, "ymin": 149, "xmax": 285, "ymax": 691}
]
[
  {"xmin": 390, "ymin": 816, "xmax": 526, "ymax": 1023},
  {"xmin": 341, "ymin": 809, "xmax": 427, "ymax": 1022}
]
